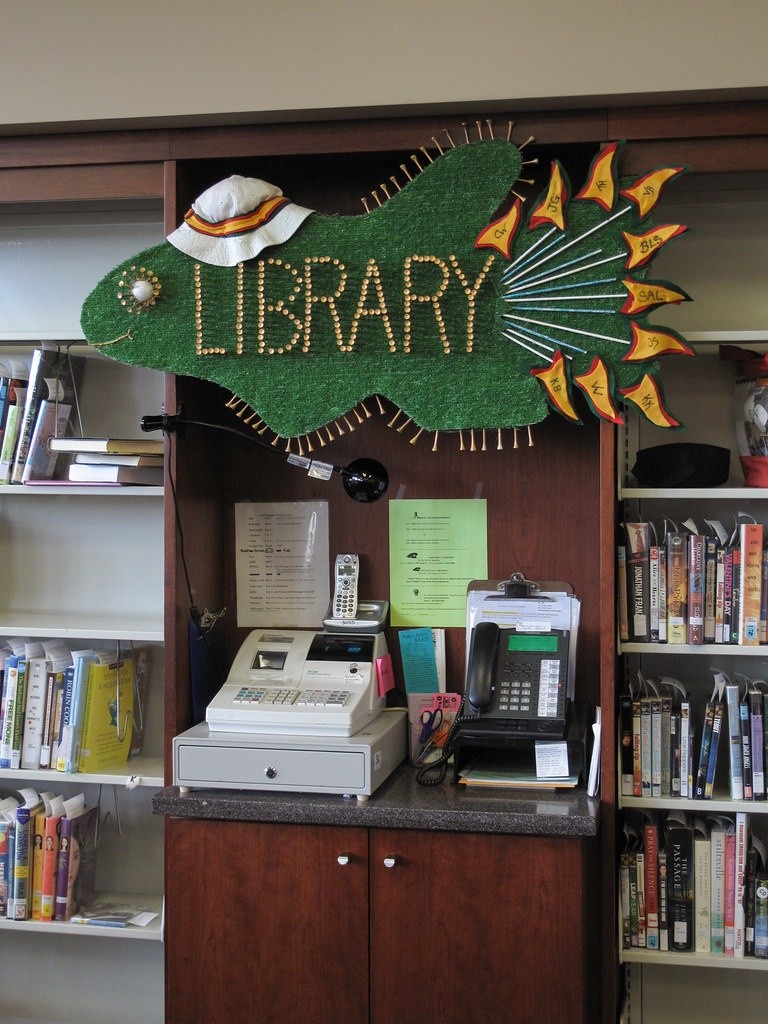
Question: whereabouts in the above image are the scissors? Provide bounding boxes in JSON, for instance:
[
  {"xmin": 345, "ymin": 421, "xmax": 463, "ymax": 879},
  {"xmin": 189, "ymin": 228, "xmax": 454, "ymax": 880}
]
[{"xmin": 408, "ymin": 708, "xmax": 452, "ymax": 769}]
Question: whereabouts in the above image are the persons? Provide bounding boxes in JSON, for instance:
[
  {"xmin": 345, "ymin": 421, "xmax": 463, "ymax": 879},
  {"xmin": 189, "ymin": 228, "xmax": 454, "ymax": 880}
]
[
  {"xmin": 45, "ymin": 835, "xmax": 54, "ymax": 852},
  {"xmin": 60, "ymin": 836, "xmax": 68, "ymax": 852},
  {"xmin": 33, "ymin": 834, "xmax": 42, "ymax": 851},
  {"xmin": 66, "ymin": 832, "xmax": 86, "ymax": 918},
  {"xmin": 659, "ymin": 864, "xmax": 666, "ymax": 878}
]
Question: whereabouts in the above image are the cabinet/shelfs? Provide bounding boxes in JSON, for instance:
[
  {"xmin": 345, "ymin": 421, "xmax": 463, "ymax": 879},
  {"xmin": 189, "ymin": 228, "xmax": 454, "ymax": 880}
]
[{"xmin": 0, "ymin": 328, "xmax": 768, "ymax": 1024}]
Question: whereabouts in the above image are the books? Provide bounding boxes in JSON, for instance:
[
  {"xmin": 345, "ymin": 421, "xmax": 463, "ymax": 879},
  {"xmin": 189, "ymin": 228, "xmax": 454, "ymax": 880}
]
[
  {"xmin": 620, "ymin": 811, "xmax": 768, "ymax": 962},
  {"xmin": 613, "ymin": 689, "xmax": 768, "ymax": 803},
  {"xmin": 615, "ymin": 522, "xmax": 768, "ymax": 647},
  {"xmin": 0, "ymin": 804, "xmax": 152, "ymax": 928},
  {"xmin": 0, "ymin": 646, "xmax": 156, "ymax": 774},
  {"xmin": 0, "ymin": 350, "xmax": 165, "ymax": 487}
]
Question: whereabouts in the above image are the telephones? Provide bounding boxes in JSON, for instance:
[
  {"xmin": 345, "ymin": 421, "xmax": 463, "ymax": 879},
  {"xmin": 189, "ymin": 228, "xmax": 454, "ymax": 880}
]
[
  {"xmin": 321, "ymin": 551, "xmax": 390, "ymax": 634},
  {"xmin": 448, "ymin": 622, "xmax": 572, "ymax": 766}
]
